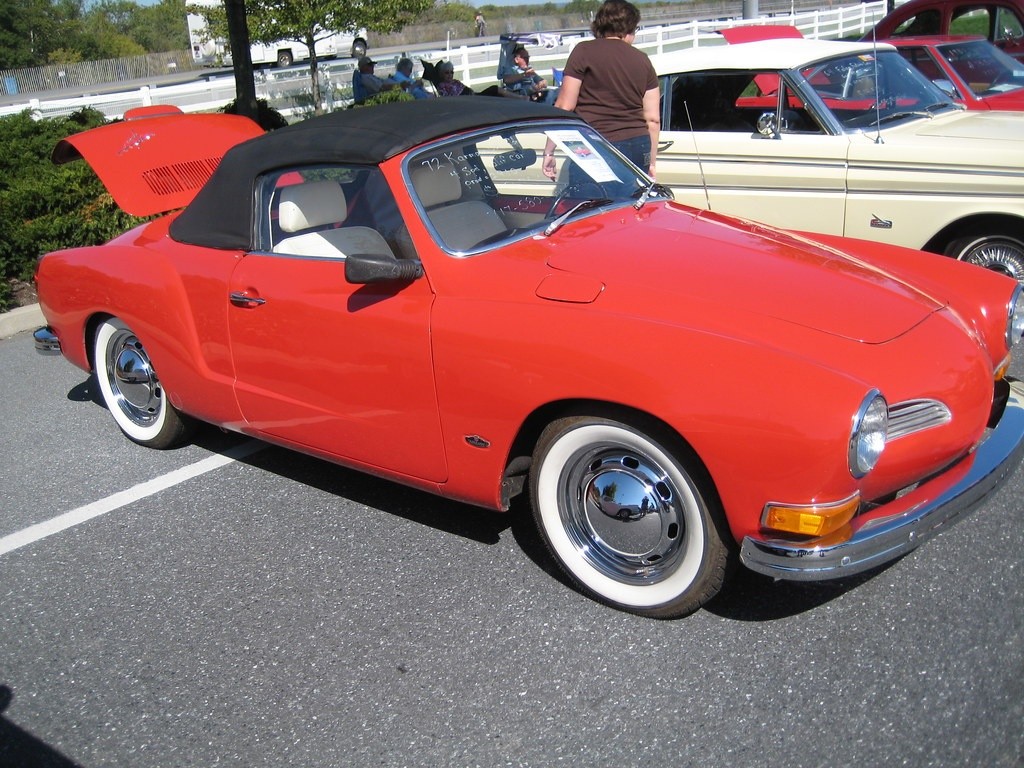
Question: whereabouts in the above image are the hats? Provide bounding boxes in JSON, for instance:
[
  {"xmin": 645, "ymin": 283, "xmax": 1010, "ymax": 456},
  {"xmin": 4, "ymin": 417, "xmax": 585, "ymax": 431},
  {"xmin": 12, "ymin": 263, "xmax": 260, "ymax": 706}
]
[
  {"xmin": 358, "ymin": 57, "xmax": 377, "ymax": 68},
  {"xmin": 515, "ymin": 48, "xmax": 530, "ymax": 58}
]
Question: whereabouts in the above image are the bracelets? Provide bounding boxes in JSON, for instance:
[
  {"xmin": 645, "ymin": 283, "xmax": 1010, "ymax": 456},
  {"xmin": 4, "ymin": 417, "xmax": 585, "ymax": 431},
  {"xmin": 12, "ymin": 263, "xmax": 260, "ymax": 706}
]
[{"xmin": 543, "ymin": 154, "xmax": 554, "ymax": 157}]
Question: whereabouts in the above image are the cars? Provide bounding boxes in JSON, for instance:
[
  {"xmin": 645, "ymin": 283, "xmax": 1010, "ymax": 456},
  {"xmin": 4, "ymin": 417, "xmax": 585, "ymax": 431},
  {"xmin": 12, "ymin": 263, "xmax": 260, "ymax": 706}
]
[
  {"xmin": 856, "ymin": 0, "xmax": 1024, "ymax": 84},
  {"xmin": 33, "ymin": 104, "xmax": 1023, "ymax": 621},
  {"xmin": 471, "ymin": 36, "xmax": 1024, "ymax": 339},
  {"xmin": 717, "ymin": 23, "xmax": 1024, "ymax": 110}
]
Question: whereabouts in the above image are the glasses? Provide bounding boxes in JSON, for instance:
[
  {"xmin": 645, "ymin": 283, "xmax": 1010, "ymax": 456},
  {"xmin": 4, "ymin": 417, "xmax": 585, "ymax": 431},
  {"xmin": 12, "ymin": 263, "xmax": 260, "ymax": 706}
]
[
  {"xmin": 443, "ymin": 71, "xmax": 454, "ymax": 74},
  {"xmin": 635, "ymin": 26, "xmax": 640, "ymax": 33}
]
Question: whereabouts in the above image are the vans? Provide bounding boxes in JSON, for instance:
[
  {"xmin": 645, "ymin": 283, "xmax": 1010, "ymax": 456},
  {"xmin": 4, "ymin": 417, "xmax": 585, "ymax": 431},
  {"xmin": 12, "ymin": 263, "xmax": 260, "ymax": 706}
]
[{"xmin": 185, "ymin": 1, "xmax": 370, "ymax": 69}]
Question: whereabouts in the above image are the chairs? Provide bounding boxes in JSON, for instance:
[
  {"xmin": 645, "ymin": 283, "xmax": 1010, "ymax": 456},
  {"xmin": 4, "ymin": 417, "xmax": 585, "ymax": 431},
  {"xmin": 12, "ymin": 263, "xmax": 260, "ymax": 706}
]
[
  {"xmin": 495, "ymin": 33, "xmax": 562, "ymax": 96},
  {"xmin": 276, "ymin": 179, "xmax": 396, "ymax": 262},
  {"xmin": 392, "ymin": 161, "xmax": 508, "ymax": 263},
  {"xmin": 419, "ymin": 58, "xmax": 444, "ymax": 96}
]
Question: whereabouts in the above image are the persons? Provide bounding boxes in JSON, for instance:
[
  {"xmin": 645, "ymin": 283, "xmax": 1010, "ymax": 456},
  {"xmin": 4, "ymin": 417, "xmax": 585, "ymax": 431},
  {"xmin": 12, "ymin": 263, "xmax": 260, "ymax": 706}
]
[
  {"xmin": 475, "ymin": 11, "xmax": 487, "ymax": 37},
  {"xmin": 394, "ymin": 58, "xmax": 437, "ymax": 100},
  {"xmin": 503, "ymin": 47, "xmax": 547, "ymax": 95},
  {"xmin": 542, "ymin": 0, "xmax": 661, "ymax": 218},
  {"xmin": 359, "ymin": 57, "xmax": 411, "ymax": 104},
  {"xmin": 437, "ymin": 61, "xmax": 546, "ymax": 101}
]
